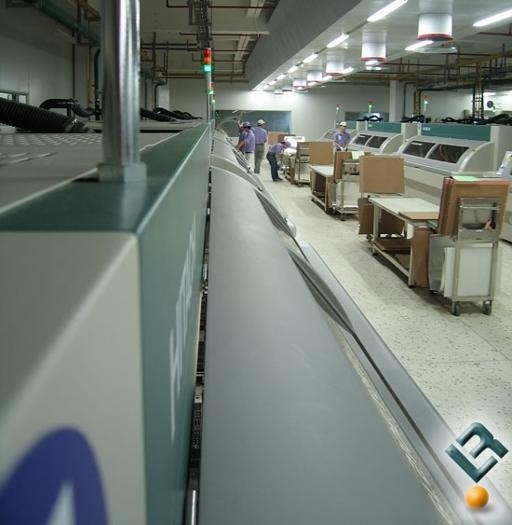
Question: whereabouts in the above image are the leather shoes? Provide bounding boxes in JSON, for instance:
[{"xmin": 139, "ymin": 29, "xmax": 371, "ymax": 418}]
[{"xmin": 273, "ymin": 177, "xmax": 282, "ymax": 181}]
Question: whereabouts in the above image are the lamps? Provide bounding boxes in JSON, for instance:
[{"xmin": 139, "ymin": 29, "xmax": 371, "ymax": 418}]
[{"xmin": 272, "ymin": 12, "xmax": 454, "ymax": 96}]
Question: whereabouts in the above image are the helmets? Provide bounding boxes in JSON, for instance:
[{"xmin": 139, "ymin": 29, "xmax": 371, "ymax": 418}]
[
  {"xmin": 257, "ymin": 120, "xmax": 266, "ymax": 126},
  {"xmin": 340, "ymin": 122, "xmax": 346, "ymax": 127},
  {"xmin": 239, "ymin": 122, "xmax": 251, "ymax": 128}
]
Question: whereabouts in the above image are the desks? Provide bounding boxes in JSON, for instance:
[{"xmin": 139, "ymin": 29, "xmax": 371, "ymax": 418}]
[
  {"xmin": 310, "ymin": 164, "xmax": 335, "ymax": 211},
  {"xmin": 367, "ymin": 195, "xmax": 441, "ymax": 288}
]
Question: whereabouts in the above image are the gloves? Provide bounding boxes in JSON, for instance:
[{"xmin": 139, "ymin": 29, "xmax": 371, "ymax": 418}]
[{"xmin": 340, "ymin": 147, "xmax": 347, "ymax": 152}]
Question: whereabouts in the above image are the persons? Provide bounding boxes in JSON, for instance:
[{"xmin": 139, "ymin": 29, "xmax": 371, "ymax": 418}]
[
  {"xmin": 234, "ymin": 122, "xmax": 247, "ymax": 156},
  {"xmin": 334, "ymin": 121, "xmax": 351, "ymax": 152},
  {"xmin": 266, "ymin": 142, "xmax": 291, "ymax": 182},
  {"xmin": 235, "ymin": 122, "xmax": 255, "ymax": 164},
  {"xmin": 253, "ymin": 120, "xmax": 267, "ymax": 173}
]
